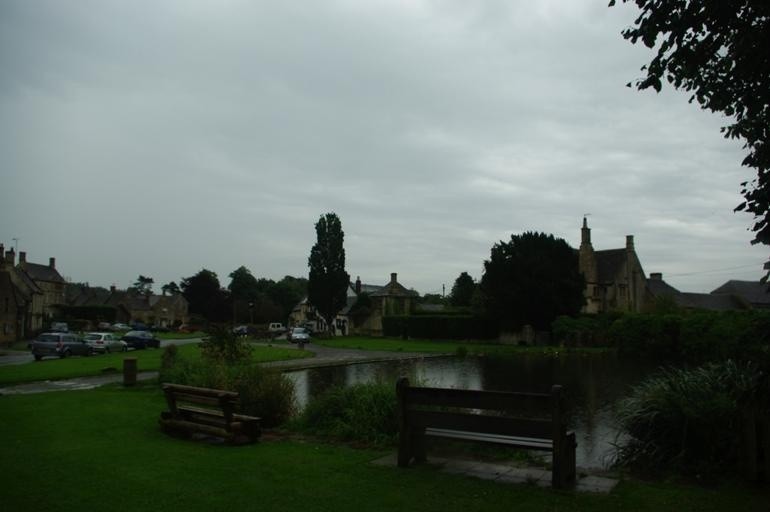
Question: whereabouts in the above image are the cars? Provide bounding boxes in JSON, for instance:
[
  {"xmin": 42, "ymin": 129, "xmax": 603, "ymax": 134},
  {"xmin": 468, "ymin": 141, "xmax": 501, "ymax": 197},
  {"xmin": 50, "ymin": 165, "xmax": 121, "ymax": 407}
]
[
  {"xmin": 120, "ymin": 331, "xmax": 161, "ymax": 350},
  {"xmin": 291, "ymin": 328, "xmax": 311, "ymax": 342},
  {"xmin": 268, "ymin": 321, "xmax": 288, "ymax": 332},
  {"xmin": 158, "ymin": 327, "xmax": 173, "ymax": 333},
  {"xmin": 287, "ymin": 327, "xmax": 296, "ymax": 343},
  {"xmin": 233, "ymin": 325, "xmax": 282, "ymax": 339},
  {"xmin": 28, "ymin": 332, "xmax": 92, "ymax": 361},
  {"xmin": 177, "ymin": 324, "xmax": 203, "ymax": 333},
  {"xmin": 113, "ymin": 322, "xmax": 132, "ymax": 331},
  {"xmin": 83, "ymin": 333, "xmax": 128, "ymax": 354},
  {"xmin": 134, "ymin": 323, "xmax": 148, "ymax": 331}
]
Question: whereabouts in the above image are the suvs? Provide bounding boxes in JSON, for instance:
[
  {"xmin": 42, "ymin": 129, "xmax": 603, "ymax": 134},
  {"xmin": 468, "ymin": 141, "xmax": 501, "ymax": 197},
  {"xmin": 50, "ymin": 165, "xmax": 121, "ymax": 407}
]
[
  {"xmin": 47, "ymin": 322, "xmax": 68, "ymax": 334},
  {"xmin": 98, "ymin": 321, "xmax": 114, "ymax": 333}
]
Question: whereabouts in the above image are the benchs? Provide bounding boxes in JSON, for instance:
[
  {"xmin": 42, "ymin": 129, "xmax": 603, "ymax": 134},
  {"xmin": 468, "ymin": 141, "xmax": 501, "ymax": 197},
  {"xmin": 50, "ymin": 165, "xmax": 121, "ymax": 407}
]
[
  {"xmin": 159, "ymin": 382, "xmax": 261, "ymax": 444},
  {"xmin": 390, "ymin": 376, "xmax": 577, "ymax": 489}
]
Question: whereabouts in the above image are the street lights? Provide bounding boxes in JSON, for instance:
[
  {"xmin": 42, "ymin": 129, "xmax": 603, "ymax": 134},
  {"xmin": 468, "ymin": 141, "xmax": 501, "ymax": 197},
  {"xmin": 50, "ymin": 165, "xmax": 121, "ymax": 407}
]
[
  {"xmin": 163, "ymin": 307, "xmax": 168, "ymax": 326},
  {"xmin": 12, "ymin": 237, "xmax": 20, "ymax": 261},
  {"xmin": 248, "ymin": 302, "xmax": 254, "ymax": 323}
]
